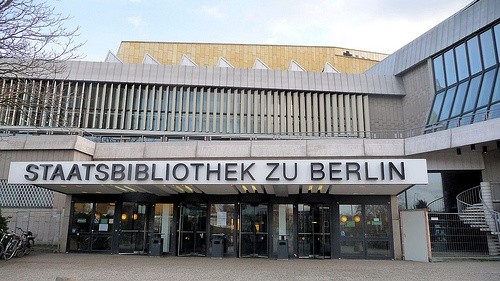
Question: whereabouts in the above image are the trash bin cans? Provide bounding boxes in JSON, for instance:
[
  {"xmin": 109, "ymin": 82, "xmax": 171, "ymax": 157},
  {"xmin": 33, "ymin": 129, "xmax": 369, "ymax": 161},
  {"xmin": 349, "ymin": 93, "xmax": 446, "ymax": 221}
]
[
  {"xmin": 211, "ymin": 233, "xmax": 225, "ymax": 258},
  {"xmin": 277, "ymin": 239, "xmax": 289, "ymax": 261},
  {"xmin": 150, "ymin": 233, "xmax": 162, "ymax": 256}
]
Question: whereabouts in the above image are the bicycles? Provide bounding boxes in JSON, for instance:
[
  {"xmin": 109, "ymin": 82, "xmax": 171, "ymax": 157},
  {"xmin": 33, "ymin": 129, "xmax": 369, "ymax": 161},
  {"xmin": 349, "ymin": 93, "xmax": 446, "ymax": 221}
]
[{"xmin": 0, "ymin": 226, "xmax": 38, "ymax": 261}]
